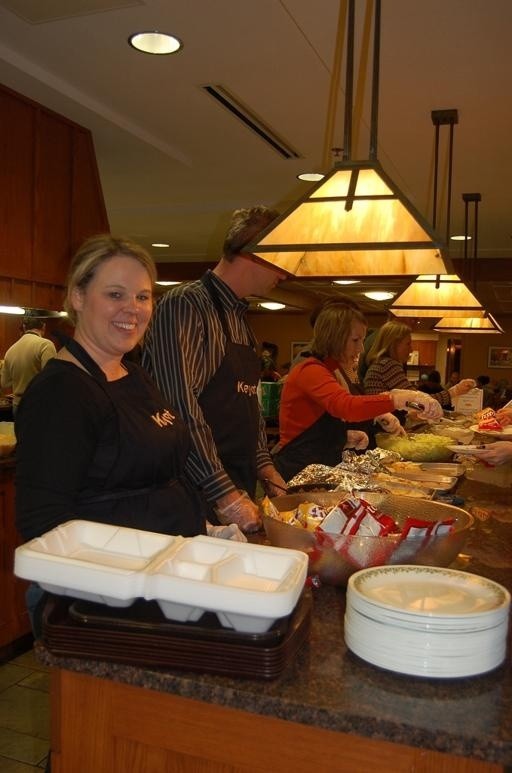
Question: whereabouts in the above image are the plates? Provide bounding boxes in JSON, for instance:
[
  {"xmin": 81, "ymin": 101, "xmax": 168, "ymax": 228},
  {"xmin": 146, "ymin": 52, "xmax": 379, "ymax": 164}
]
[
  {"xmin": 344, "ymin": 565, "xmax": 509, "ymax": 682},
  {"xmin": 446, "ymin": 411, "xmax": 512, "ymax": 457}
]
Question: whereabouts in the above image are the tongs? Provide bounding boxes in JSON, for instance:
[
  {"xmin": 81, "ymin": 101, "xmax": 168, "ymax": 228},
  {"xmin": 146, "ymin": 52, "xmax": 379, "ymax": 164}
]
[{"xmin": 407, "ymin": 401, "xmax": 457, "ymax": 427}]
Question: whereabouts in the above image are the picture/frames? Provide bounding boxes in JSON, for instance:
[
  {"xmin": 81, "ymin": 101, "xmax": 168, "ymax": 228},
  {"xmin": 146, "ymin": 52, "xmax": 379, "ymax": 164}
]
[
  {"xmin": 289, "ymin": 340, "xmax": 313, "ymax": 362},
  {"xmin": 486, "ymin": 343, "xmax": 512, "ymax": 369}
]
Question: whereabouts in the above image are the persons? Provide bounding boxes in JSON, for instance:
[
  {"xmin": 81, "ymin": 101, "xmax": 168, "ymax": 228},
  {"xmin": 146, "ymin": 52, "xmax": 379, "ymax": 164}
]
[
  {"xmin": 415, "ymin": 371, "xmax": 444, "ymax": 395},
  {"xmin": 278, "ymin": 361, "xmax": 292, "ymax": 381},
  {"xmin": 476, "ymin": 374, "xmax": 497, "ymax": 408},
  {"xmin": 139, "ymin": 203, "xmax": 290, "ymax": 532},
  {"xmin": 444, "ymin": 370, "xmax": 461, "ymax": 388},
  {"xmin": 497, "ymin": 375, "xmax": 511, "ymax": 410},
  {"xmin": 17, "ymin": 234, "xmax": 247, "ymax": 544},
  {"xmin": 260, "ymin": 351, "xmax": 279, "ymax": 381},
  {"xmin": 272, "ymin": 302, "xmax": 444, "ymax": 484},
  {"xmin": 310, "ymin": 294, "xmax": 407, "ymax": 455},
  {"xmin": 363, "ymin": 320, "xmax": 476, "ymax": 419},
  {"xmin": 3, "ymin": 317, "xmax": 61, "ymax": 412}
]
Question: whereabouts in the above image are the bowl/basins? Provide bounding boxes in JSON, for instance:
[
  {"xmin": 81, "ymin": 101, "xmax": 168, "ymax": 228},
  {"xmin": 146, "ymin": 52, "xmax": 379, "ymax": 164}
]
[
  {"xmin": 377, "ymin": 433, "xmax": 455, "ymax": 460},
  {"xmin": 260, "ymin": 491, "xmax": 474, "ymax": 586}
]
